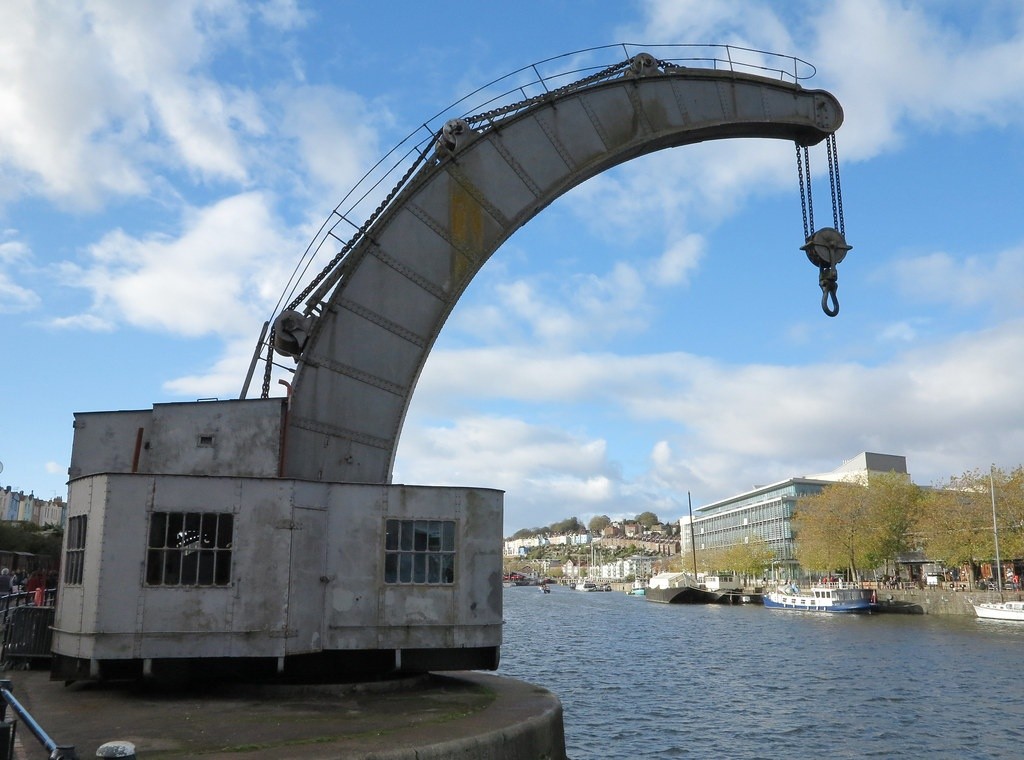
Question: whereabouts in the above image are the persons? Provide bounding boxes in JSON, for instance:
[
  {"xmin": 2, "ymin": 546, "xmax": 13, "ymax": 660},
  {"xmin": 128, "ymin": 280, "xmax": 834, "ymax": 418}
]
[
  {"xmin": 0, "ymin": 567, "xmax": 59, "ymax": 617},
  {"xmin": 823, "ymin": 575, "xmax": 837, "ymax": 588},
  {"xmin": 946, "ymin": 572, "xmax": 967, "ymax": 588},
  {"xmin": 882, "ymin": 574, "xmax": 904, "ymax": 589}
]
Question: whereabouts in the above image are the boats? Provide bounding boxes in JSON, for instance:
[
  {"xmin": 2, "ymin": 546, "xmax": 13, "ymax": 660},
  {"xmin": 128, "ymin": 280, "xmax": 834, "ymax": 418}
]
[
  {"xmin": 758, "ymin": 568, "xmax": 882, "ymax": 614},
  {"xmin": 627, "ymin": 588, "xmax": 646, "ymax": 596},
  {"xmin": 538, "ymin": 584, "xmax": 550, "ymax": 594},
  {"xmin": 503, "ymin": 570, "xmax": 559, "ymax": 587}
]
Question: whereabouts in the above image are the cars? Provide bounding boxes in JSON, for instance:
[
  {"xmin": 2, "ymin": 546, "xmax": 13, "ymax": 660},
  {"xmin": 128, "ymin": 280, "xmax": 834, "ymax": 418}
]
[{"xmin": 975, "ymin": 576, "xmax": 1023, "ymax": 590}]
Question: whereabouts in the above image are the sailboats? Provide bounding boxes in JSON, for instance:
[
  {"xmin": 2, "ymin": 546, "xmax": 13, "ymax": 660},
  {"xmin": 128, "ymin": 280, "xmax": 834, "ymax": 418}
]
[
  {"xmin": 570, "ymin": 547, "xmax": 613, "ymax": 594},
  {"xmin": 972, "ymin": 464, "xmax": 1024, "ymax": 620}
]
